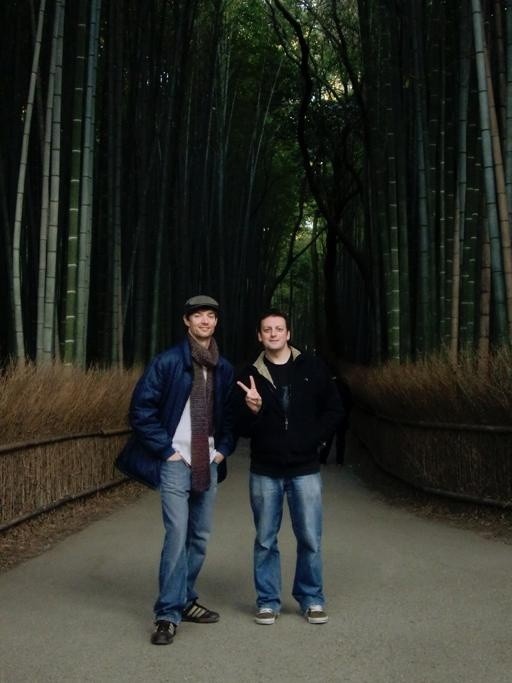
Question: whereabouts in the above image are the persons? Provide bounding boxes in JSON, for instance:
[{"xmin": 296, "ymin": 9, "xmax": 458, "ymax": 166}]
[
  {"xmin": 112, "ymin": 294, "xmax": 247, "ymax": 645},
  {"xmin": 318, "ymin": 364, "xmax": 355, "ymax": 467},
  {"xmin": 223, "ymin": 309, "xmax": 344, "ymax": 625}
]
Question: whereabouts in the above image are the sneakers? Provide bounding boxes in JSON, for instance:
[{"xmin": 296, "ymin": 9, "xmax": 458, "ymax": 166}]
[
  {"xmin": 181, "ymin": 602, "xmax": 220, "ymax": 624},
  {"xmin": 254, "ymin": 607, "xmax": 281, "ymax": 625},
  {"xmin": 300, "ymin": 603, "xmax": 328, "ymax": 624},
  {"xmin": 150, "ymin": 619, "xmax": 178, "ymax": 644}
]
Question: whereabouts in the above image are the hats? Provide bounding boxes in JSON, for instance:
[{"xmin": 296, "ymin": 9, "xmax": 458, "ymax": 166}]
[{"xmin": 182, "ymin": 293, "xmax": 221, "ymax": 317}]
